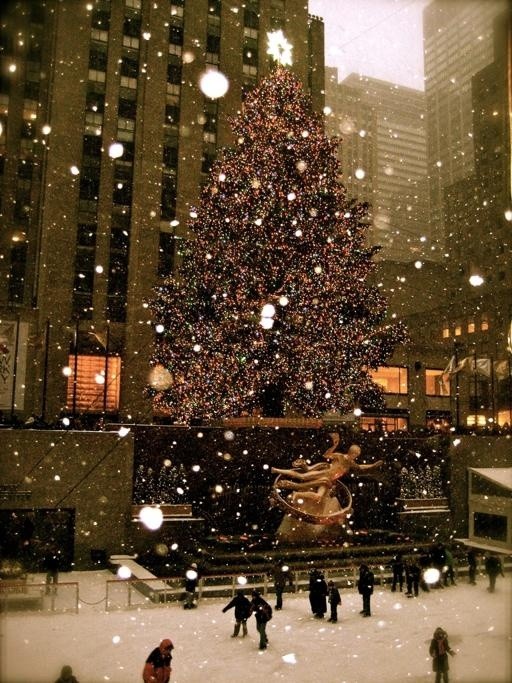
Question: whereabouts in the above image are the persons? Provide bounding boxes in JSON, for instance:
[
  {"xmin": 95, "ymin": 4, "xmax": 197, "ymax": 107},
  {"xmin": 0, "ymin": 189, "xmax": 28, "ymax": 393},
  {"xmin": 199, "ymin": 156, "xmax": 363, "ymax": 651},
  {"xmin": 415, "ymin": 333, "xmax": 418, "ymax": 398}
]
[
  {"xmin": 143, "ymin": 639, "xmax": 173, "ymax": 683},
  {"xmin": 307, "ymin": 569, "xmax": 329, "ymax": 618},
  {"xmin": 389, "ymin": 542, "xmax": 502, "ymax": 596},
  {"xmin": 270, "ymin": 432, "xmax": 383, "ymax": 502},
  {"xmin": 357, "ymin": 564, "xmax": 377, "ymax": 618},
  {"xmin": 429, "ymin": 627, "xmax": 456, "ymax": 683},
  {"xmin": 251, "ymin": 590, "xmax": 271, "ymax": 650},
  {"xmin": 55, "ymin": 665, "xmax": 78, "ymax": 682},
  {"xmin": 44, "ymin": 543, "xmax": 64, "ymax": 595},
  {"xmin": 270, "ymin": 556, "xmax": 295, "ymax": 610},
  {"xmin": 183, "ymin": 563, "xmax": 200, "ymax": 608},
  {"xmin": 223, "ymin": 591, "xmax": 250, "ymax": 643},
  {"xmin": 327, "ymin": 579, "xmax": 340, "ymax": 624}
]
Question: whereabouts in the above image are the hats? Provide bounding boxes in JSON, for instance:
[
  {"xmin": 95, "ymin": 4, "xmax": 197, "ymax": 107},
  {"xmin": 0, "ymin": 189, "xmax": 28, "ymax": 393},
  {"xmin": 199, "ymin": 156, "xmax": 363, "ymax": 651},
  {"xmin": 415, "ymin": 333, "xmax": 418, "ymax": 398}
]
[
  {"xmin": 484, "ymin": 551, "xmax": 491, "ymax": 559},
  {"xmin": 328, "ymin": 580, "xmax": 334, "ymax": 586}
]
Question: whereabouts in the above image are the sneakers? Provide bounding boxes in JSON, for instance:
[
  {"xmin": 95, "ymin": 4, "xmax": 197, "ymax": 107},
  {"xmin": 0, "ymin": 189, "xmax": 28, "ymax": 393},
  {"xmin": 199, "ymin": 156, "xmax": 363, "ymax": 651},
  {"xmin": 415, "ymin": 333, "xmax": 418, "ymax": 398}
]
[
  {"xmin": 315, "ymin": 613, "xmax": 338, "ymax": 624},
  {"xmin": 467, "ymin": 580, "xmax": 495, "ymax": 594},
  {"xmin": 359, "ymin": 610, "xmax": 370, "ymax": 616},
  {"xmin": 391, "ymin": 580, "xmax": 457, "ymax": 598}
]
